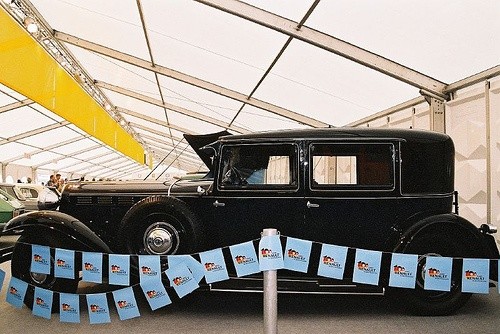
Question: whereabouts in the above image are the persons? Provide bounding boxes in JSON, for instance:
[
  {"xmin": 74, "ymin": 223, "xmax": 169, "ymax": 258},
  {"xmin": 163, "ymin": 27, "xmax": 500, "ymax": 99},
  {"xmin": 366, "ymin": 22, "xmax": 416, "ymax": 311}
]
[{"xmin": 17, "ymin": 173, "xmax": 123, "ymax": 194}]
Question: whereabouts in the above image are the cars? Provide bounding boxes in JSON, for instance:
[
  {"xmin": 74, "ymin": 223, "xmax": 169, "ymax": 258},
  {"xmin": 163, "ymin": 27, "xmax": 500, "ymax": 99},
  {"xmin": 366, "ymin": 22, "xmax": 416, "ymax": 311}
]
[
  {"xmin": 0, "ymin": 183, "xmax": 61, "ymax": 225},
  {"xmin": 0, "ymin": 126, "xmax": 500, "ymax": 317}
]
